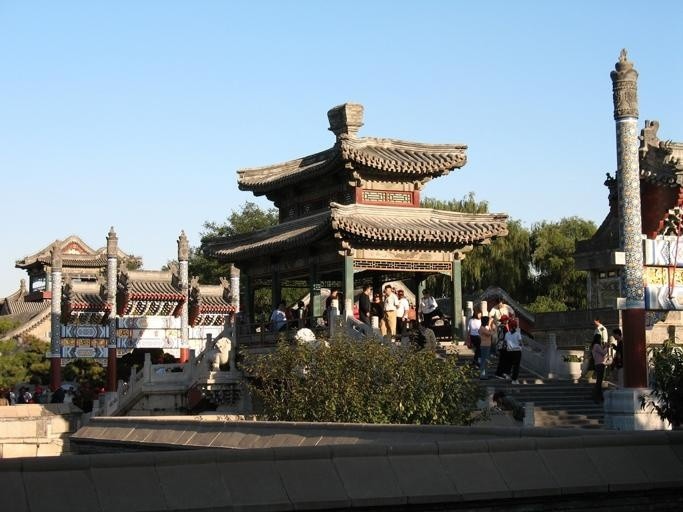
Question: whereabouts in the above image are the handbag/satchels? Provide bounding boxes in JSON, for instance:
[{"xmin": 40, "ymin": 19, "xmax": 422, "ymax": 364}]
[
  {"xmin": 588, "ymin": 358, "xmax": 595, "ymax": 370},
  {"xmin": 467, "ymin": 335, "xmax": 473, "ymax": 348}
]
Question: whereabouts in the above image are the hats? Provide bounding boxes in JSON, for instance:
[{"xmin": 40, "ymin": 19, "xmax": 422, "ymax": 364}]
[
  {"xmin": 501, "ymin": 315, "xmax": 509, "ymax": 320},
  {"xmin": 511, "ymin": 320, "xmax": 517, "ymax": 326}
]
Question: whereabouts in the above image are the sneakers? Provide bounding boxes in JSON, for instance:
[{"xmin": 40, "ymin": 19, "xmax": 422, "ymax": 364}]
[{"xmin": 496, "ymin": 373, "xmax": 520, "ymax": 385}]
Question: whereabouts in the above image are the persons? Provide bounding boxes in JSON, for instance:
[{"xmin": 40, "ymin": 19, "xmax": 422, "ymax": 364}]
[
  {"xmin": 466, "ymin": 296, "xmax": 524, "ymax": 386},
  {"xmin": 152, "ymin": 351, "xmax": 183, "ymax": 376},
  {"xmin": 230, "ymin": 298, "xmax": 311, "ymax": 334},
  {"xmin": 580, "ymin": 316, "xmax": 623, "ymax": 402},
  {"xmin": 0, "ymin": 368, "xmax": 108, "ymax": 414},
  {"xmin": 323, "ymin": 283, "xmax": 448, "ymax": 342}
]
